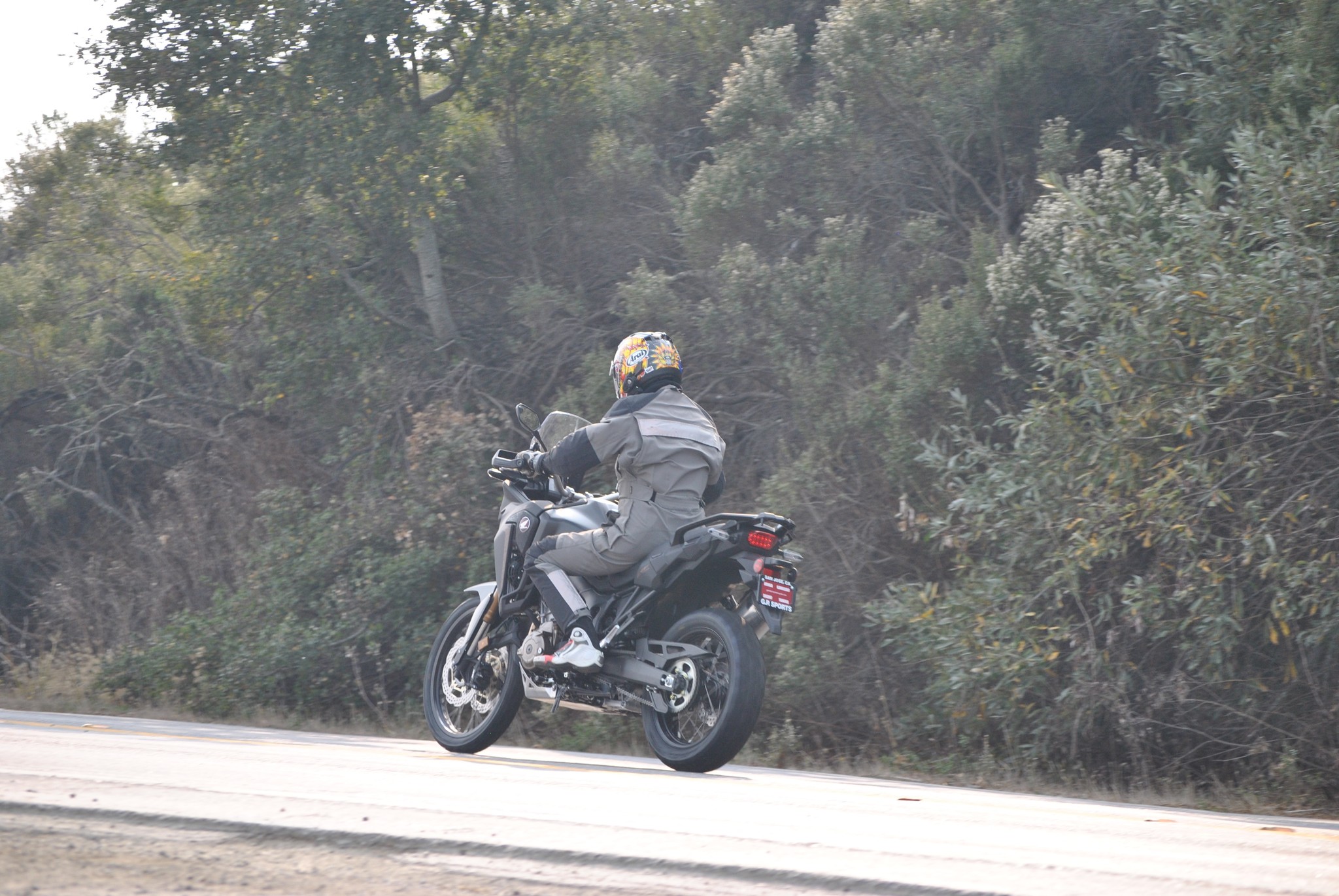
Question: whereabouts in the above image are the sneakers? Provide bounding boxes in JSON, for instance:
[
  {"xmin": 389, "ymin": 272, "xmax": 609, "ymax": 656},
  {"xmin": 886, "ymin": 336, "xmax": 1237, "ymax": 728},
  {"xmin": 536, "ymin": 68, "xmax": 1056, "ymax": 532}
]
[{"xmin": 535, "ymin": 627, "xmax": 604, "ymax": 674}]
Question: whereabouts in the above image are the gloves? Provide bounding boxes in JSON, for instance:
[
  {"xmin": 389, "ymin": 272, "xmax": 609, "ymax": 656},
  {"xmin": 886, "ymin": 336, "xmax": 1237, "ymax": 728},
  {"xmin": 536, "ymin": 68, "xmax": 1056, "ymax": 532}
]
[{"xmin": 515, "ymin": 450, "xmax": 548, "ymax": 481}]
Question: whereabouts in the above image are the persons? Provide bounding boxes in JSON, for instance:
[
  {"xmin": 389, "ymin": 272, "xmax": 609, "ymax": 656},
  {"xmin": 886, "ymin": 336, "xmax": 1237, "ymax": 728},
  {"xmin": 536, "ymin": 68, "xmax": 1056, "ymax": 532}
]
[{"xmin": 515, "ymin": 327, "xmax": 730, "ymax": 689}]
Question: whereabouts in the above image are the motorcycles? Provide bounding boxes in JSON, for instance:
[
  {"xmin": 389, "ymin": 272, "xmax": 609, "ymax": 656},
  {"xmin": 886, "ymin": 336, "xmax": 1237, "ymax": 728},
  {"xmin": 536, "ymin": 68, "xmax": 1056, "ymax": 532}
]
[{"xmin": 419, "ymin": 397, "xmax": 804, "ymax": 772}]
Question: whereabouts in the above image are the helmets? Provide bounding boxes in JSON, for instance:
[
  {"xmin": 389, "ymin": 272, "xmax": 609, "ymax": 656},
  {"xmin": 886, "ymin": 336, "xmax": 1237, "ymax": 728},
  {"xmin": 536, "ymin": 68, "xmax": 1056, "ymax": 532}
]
[{"xmin": 608, "ymin": 331, "xmax": 683, "ymax": 399}]
